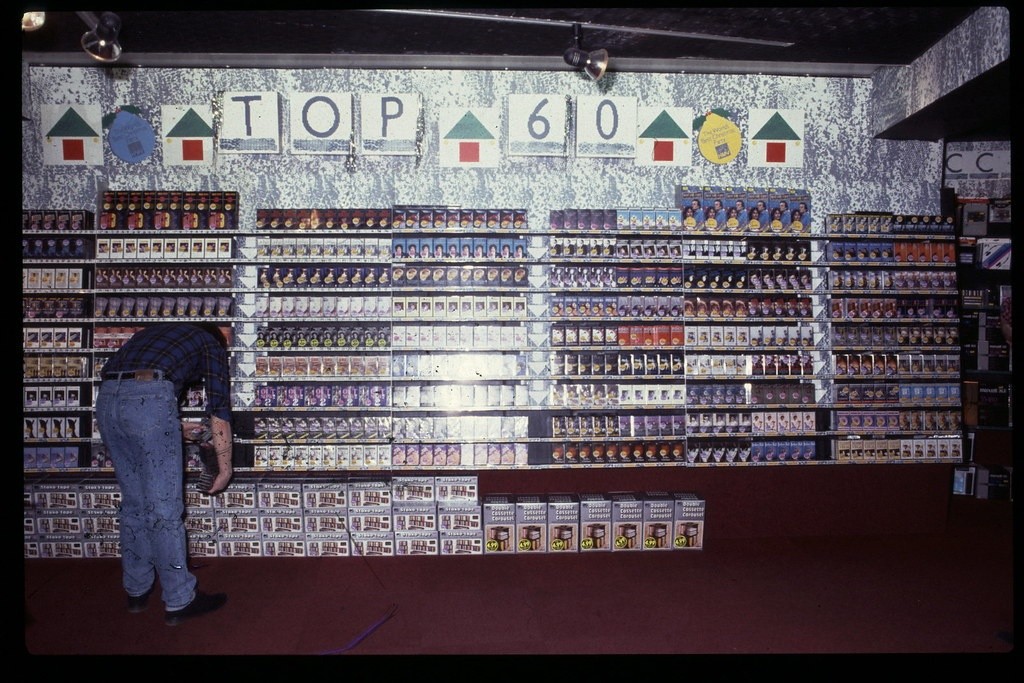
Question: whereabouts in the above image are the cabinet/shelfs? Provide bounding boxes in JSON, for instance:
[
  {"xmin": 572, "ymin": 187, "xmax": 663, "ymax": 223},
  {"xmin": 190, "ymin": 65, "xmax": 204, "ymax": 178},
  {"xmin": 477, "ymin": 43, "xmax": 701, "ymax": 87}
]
[{"xmin": 18, "ymin": 218, "xmax": 974, "ymax": 472}]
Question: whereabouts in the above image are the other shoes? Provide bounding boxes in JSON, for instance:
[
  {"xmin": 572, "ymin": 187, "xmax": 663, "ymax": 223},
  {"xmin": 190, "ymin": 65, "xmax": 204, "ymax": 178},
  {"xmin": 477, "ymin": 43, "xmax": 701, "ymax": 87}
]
[
  {"xmin": 164, "ymin": 589, "xmax": 228, "ymax": 626},
  {"xmin": 128, "ymin": 583, "xmax": 155, "ymax": 612}
]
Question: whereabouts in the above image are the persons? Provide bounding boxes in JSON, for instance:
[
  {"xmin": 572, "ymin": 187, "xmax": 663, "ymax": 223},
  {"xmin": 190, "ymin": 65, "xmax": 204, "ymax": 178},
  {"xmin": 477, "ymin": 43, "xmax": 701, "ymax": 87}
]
[
  {"xmin": 682, "ymin": 199, "xmax": 811, "ymax": 233},
  {"xmin": 94, "ymin": 323, "xmax": 233, "ymax": 621}
]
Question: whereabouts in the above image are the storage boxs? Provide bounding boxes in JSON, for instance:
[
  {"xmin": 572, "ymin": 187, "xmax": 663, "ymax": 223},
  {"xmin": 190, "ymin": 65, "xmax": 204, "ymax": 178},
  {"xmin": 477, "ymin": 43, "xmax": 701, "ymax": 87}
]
[{"xmin": 23, "ymin": 469, "xmax": 704, "ymax": 559}]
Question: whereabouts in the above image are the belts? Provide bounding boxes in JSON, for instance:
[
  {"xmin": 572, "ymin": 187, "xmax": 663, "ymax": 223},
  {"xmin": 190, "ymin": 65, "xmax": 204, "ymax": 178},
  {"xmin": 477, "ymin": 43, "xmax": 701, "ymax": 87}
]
[{"xmin": 103, "ymin": 370, "xmax": 170, "ymax": 380}]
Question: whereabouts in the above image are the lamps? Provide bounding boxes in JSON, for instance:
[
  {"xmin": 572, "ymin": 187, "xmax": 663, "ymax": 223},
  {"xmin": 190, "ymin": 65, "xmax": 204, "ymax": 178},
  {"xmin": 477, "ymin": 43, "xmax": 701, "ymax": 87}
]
[
  {"xmin": 73, "ymin": 10, "xmax": 123, "ymax": 61},
  {"xmin": 563, "ymin": 24, "xmax": 609, "ymax": 81}
]
